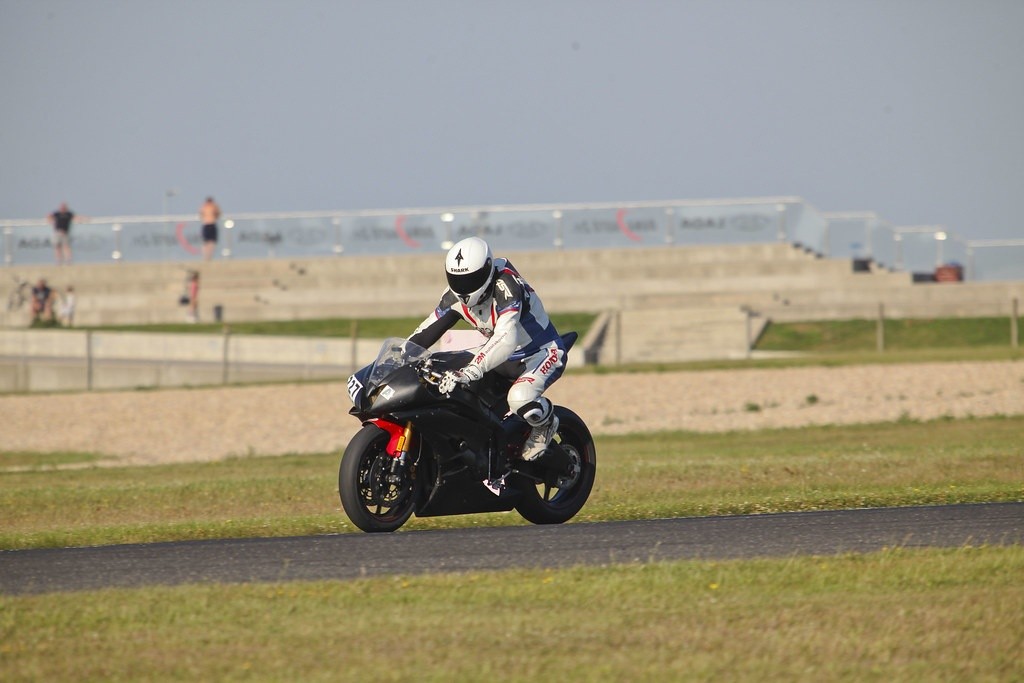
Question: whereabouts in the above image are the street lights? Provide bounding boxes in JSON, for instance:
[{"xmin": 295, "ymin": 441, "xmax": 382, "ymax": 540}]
[
  {"xmin": 934, "ymin": 233, "xmax": 975, "ymax": 282},
  {"xmin": 161, "ymin": 191, "xmax": 174, "ymax": 256}
]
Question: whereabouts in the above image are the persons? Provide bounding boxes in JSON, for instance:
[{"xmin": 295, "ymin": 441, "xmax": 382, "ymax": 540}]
[
  {"xmin": 33, "ymin": 277, "xmax": 75, "ymax": 328},
  {"xmin": 48, "ymin": 204, "xmax": 74, "ymax": 264},
  {"xmin": 199, "ymin": 198, "xmax": 219, "ymax": 261},
  {"xmin": 185, "ymin": 271, "xmax": 200, "ymax": 324},
  {"xmin": 400, "ymin": 236, "xmax": 568, "ymax": 462}
]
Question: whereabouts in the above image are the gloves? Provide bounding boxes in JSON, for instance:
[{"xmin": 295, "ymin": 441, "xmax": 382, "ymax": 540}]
[{"xmin": 438, "ymin": 369, "xmax": 471, "ymax": 399}]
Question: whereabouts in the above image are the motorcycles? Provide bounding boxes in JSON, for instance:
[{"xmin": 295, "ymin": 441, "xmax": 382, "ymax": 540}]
[{"xmin": 338, "ymin": 331, "xmax": 596, "ymax": 533}]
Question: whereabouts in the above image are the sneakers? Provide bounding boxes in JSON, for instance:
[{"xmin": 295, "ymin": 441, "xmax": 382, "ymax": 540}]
[{"xmin": 518, "ymin": 414, "xmax": 560, "ymax": 463}]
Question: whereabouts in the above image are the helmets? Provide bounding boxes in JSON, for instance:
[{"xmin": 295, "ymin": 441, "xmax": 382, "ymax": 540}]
[{"xmin": 445, "ymin": 237, "xmax": 494, "ymax": 308}]
